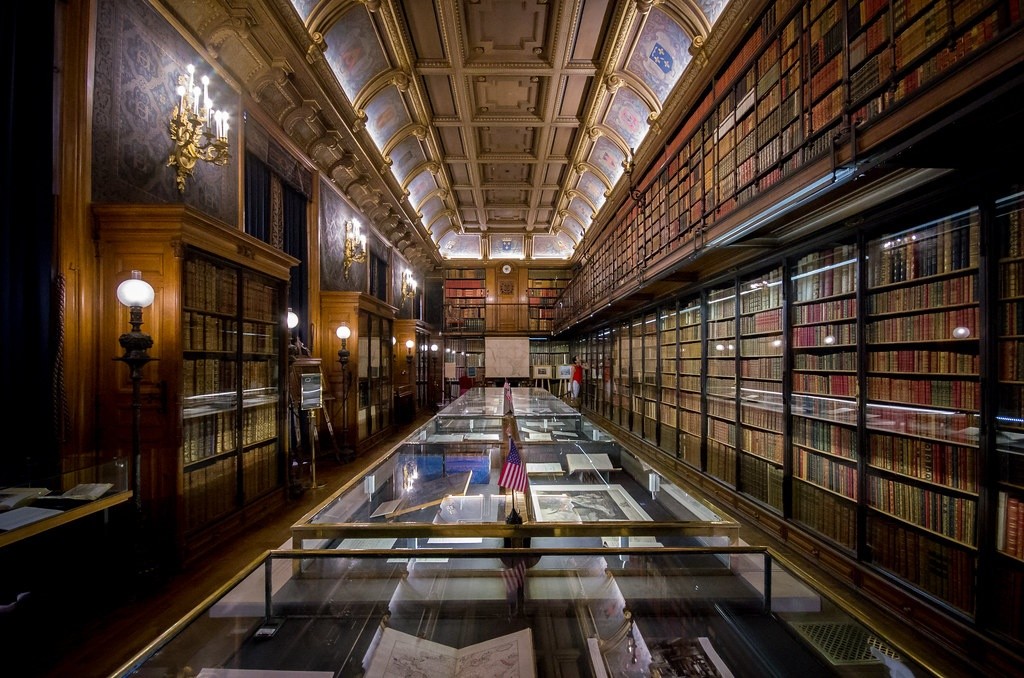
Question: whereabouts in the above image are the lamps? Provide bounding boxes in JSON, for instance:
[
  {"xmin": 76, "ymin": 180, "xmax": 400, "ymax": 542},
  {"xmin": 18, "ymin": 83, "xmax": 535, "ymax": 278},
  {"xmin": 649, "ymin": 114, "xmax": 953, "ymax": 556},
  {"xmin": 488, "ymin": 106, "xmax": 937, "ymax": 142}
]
[
  {"xmin": 431, "ymin": 344, "xmax": 439, "ymax": 381},
  {"xmin": 406, "ymin": 340, "xmax": 415, "ymax": 384},
  {"xmin": 288, "ymin": 307, "xmax": 301, "ymax": 344},
  {"xmin": 401, "ymin": 272, "xmax": 415, "ymax": 307},
  {"xmin": 336, "ymin": 322, "xmax": 355, "ymax": 456},
  {"xmin": 111, "ymin": 270, "xmax": 161, "ymax": 512},
  {"xmin": 338, "ymin": 213, "xmax": 369, "ymax": 281},
  {"xmin": 165, "ymin": 63, "xmax": 233, "ymax": 193}
]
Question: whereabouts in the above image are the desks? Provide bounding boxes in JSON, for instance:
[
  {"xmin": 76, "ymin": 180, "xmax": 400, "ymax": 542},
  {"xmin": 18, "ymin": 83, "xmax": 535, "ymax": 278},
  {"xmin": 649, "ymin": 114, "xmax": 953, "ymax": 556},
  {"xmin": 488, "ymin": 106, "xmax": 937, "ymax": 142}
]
[{"xmin": 0, "ymin": 489, "xmax": 132, "ymax": 548}]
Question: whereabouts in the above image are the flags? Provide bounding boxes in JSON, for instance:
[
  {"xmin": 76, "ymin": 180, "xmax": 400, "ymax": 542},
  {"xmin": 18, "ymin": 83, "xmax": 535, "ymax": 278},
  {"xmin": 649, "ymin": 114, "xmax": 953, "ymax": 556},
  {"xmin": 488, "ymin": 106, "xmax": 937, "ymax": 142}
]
[
  {"xmin": 504, "ymin": 381, "xmax": 512, "ymax": 400},
  {"xmin": 501, "ymin": 560, "xmax": 527, "ymax": 596},
  {"xmin": 497, "ymin": 440, "xmax": 529, "ymax": 496}
]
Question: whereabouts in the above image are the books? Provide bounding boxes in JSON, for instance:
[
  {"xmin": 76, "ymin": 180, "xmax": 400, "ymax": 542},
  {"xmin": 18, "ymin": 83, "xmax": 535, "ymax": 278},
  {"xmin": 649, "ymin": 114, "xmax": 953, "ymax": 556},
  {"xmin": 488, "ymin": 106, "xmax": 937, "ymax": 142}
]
[
  {"xmin": 441, "ymin": 0, "xmax": 1024, "ymax": 662},
  {"xmin": 181, "ymin": 256, "xmax": 278, "ymax": 461}
]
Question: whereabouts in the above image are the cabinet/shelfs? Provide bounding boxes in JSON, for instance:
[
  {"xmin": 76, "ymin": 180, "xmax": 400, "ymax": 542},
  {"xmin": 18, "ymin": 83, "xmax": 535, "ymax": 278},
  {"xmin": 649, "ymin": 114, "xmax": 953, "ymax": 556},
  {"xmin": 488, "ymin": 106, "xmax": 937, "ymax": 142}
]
[
  {"xmin": 545, "ymin": 0, "xmax": 1024, "ymax": 678},
  {"xmin": 320, "ymin": 288, "xmax": 398, "ymax": 455},
  {"xmin": 393, "ymin": 319, "xmax": 435, "ymax": 412},
  {"xmin": 94, "ymin": 204, "xmax": 300, "ymax": 568}
]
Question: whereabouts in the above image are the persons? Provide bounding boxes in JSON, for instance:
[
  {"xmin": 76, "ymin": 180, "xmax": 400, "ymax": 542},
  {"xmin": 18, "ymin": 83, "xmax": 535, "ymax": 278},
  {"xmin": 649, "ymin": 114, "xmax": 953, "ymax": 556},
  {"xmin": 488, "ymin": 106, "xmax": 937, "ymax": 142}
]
[{"xmin": 567, "ymin": 356, "xmax": 588, "ymax": 399}]
